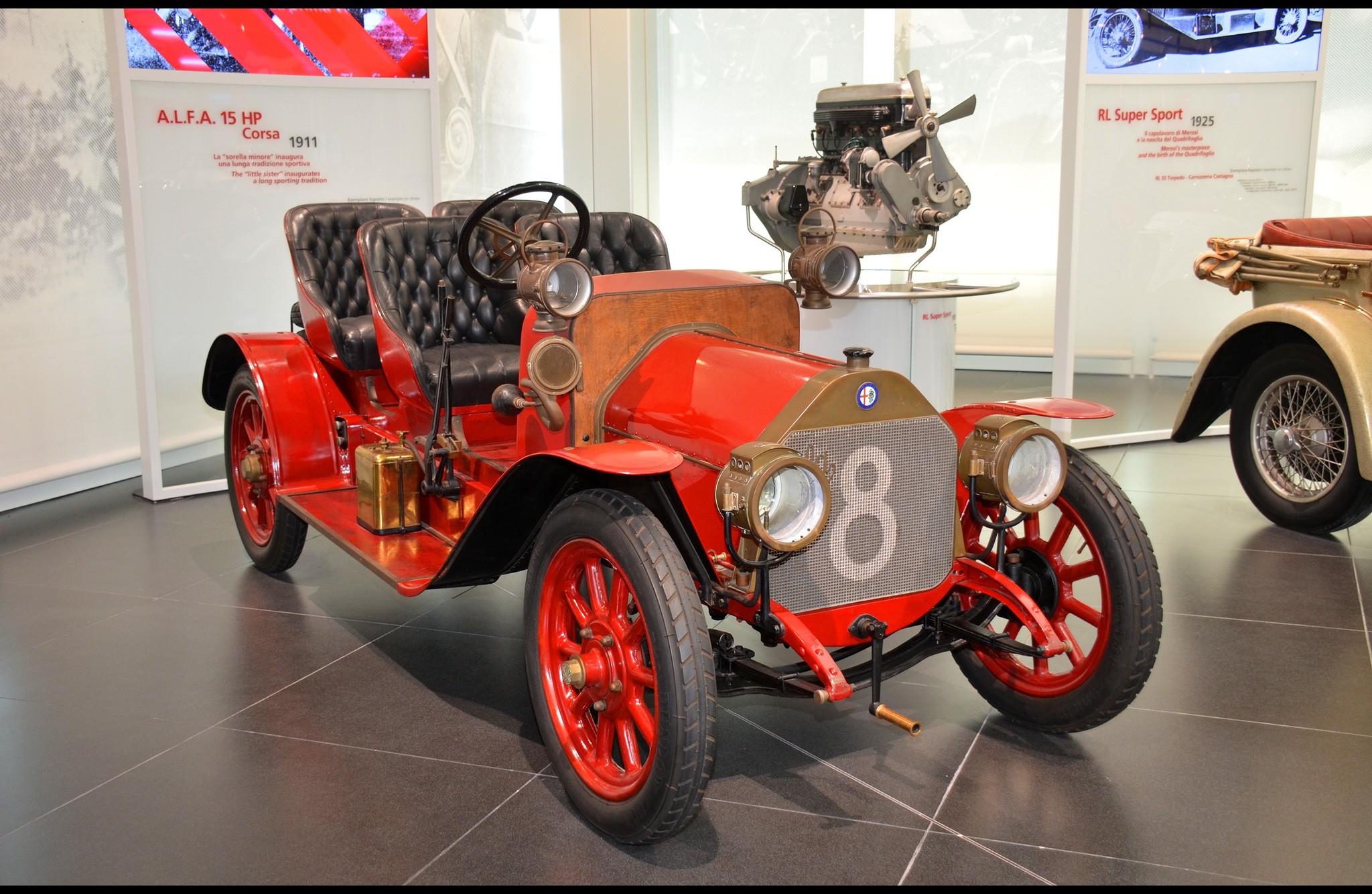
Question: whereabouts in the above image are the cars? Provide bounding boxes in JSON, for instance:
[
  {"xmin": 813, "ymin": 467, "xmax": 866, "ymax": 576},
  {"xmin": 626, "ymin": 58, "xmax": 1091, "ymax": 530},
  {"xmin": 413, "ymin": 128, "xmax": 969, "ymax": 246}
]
[
  {"xmin": 1086, "ymin": 7, "xmax": 1323, "ymax": 69},
  {"xmin": 199, "ymin": 178, "xmax": 1165, "ymax": 848},
  {"xmin": 1168, "ymin": 214, "xmax": 1372, "ymax": 536}
]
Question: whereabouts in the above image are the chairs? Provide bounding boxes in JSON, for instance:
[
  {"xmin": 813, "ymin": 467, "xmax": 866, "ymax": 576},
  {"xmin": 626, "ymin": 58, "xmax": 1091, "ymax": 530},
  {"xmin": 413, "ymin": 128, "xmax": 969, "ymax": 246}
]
[{"xmin": 283, "ymin": 200, "xmax": 671, "ymax": 417}]
